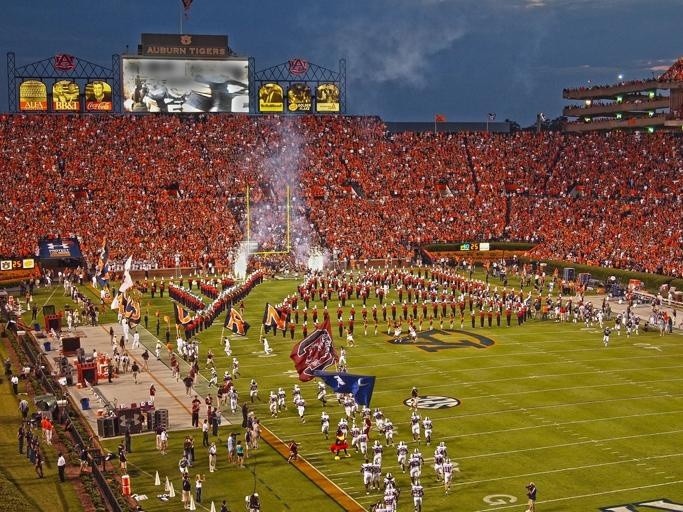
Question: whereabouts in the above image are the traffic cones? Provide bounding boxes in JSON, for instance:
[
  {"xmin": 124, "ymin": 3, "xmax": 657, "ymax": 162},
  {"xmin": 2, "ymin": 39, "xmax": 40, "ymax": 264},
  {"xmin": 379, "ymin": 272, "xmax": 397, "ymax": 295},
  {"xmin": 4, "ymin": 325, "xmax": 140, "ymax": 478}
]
[{"xmin": 153, "ymin": 471, "xmax": 215, "ymax": 512}]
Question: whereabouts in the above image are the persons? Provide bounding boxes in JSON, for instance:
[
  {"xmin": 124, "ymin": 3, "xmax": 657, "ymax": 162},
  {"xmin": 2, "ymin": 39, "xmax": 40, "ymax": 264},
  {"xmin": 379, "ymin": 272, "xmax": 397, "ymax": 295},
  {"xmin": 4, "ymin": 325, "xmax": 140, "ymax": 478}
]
[{"xmin": 1, "ymin": 56, "xmax": 683, "ymax": 511}]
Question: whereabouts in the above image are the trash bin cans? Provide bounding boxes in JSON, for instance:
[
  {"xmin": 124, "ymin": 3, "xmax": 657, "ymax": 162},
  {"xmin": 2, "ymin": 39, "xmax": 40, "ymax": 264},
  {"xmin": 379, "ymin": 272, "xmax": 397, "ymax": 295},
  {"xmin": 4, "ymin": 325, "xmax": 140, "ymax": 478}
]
[
  {"xmin": 34, "ymin": 323, "xmax": 40, "ymax": 331},
  {"xmin": 44, "ymin": 342, "xmax": 50, "ymax": 351},
  {"xmin": 80, "ymin": 397, "xmax": 90, "ymax": 410}
]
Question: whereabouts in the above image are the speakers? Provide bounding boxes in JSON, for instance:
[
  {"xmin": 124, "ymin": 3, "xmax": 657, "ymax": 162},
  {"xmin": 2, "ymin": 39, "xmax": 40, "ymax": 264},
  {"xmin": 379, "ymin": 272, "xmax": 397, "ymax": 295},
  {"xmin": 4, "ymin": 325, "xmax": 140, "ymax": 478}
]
[{"xmin": 97, "ymin": 416, "xmax": 119, "ymax": 438}]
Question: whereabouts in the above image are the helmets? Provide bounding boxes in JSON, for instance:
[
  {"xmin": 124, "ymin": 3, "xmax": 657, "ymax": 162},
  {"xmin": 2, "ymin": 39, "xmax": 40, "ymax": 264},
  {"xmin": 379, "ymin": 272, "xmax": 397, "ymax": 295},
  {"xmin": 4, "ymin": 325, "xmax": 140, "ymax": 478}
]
[{"xmin": 206, "ymin": 359, "xmax": 452, "ymax": 512}]
